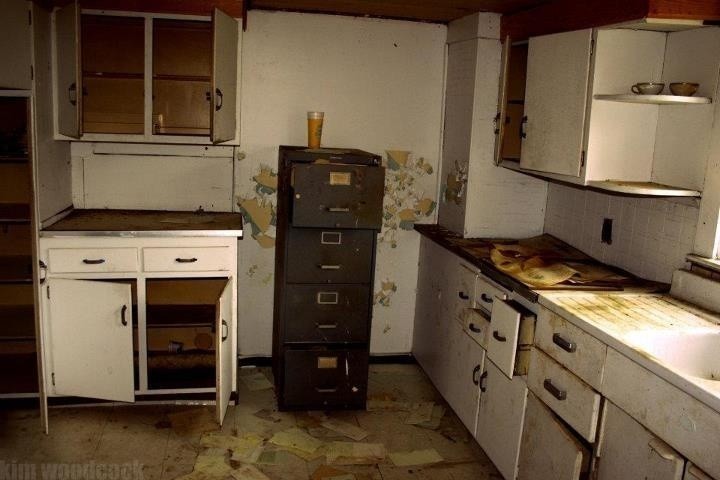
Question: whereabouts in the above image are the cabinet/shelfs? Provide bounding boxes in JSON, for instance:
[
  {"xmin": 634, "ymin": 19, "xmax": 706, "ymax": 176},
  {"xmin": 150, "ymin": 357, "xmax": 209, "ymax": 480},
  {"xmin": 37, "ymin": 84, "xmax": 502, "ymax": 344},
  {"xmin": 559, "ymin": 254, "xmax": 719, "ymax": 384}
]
[
  {"xmin": 495, "ymin": 0, "xmax": 720, "ymax": 200},
  {"xmin": 272, "ymin": 145, "xmax": 385, "ymax": 410}
]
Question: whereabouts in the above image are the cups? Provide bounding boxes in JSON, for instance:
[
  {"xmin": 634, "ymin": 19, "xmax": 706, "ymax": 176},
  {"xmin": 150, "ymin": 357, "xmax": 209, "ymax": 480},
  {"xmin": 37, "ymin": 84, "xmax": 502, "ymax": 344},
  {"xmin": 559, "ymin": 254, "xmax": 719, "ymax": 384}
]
[
  {"xmin": 631, "ymin": 81, "xmax": 665, "ymax": 95},
  {"xmin": 307, "ymin": 111, "xmax": 325, "ymax": 148}
]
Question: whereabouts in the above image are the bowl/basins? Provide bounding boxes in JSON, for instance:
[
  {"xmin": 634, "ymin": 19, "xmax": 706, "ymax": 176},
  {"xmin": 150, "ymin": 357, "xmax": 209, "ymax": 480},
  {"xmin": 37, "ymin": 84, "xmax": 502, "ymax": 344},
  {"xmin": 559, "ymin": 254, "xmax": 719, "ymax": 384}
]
[{"xmin": 668, "ymin": 81, "xmax": 701, "ymax": 97}]
